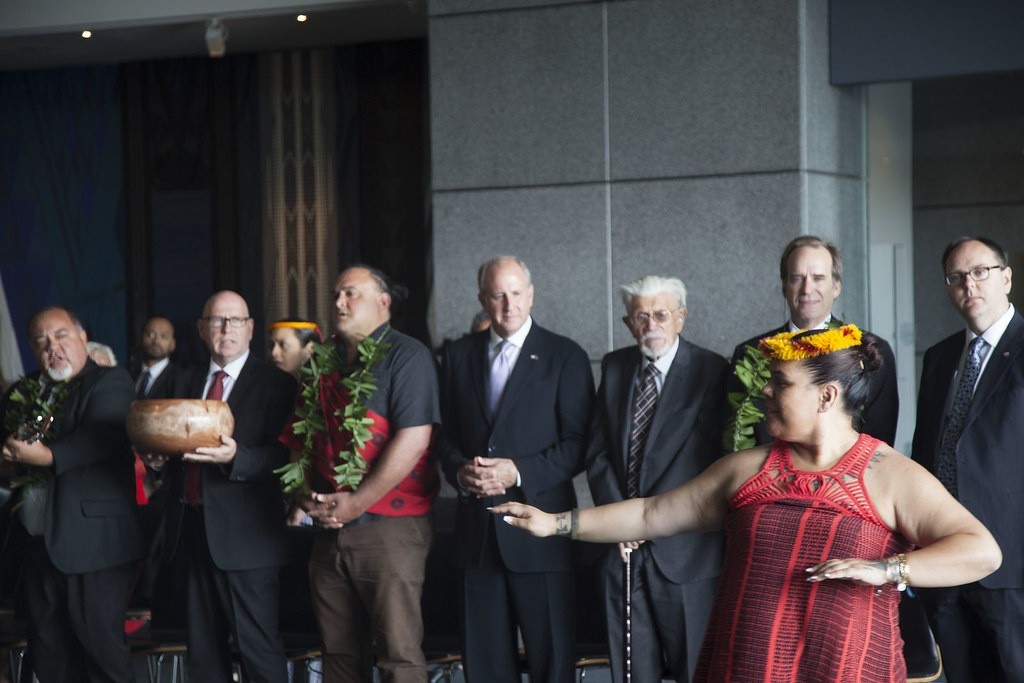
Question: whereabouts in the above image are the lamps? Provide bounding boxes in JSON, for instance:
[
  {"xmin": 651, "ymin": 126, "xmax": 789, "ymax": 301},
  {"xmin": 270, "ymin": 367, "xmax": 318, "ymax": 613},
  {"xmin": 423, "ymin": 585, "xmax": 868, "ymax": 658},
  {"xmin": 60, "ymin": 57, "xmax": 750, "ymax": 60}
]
[{"xmin": 204, "ymin": 27, "xmax": 227, "ymax": 57}]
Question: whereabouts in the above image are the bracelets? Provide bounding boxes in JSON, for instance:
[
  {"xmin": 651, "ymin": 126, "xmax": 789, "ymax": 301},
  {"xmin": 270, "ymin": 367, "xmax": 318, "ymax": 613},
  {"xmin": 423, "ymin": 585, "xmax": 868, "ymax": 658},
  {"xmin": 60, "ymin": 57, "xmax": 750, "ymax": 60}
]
[
  {"xmin": 570, "ymin": 508, "xmax": 579, "ymax": 538},
  {"xmin": 897, "ymin": 553, "xmax": 910, "ymax": 591}
]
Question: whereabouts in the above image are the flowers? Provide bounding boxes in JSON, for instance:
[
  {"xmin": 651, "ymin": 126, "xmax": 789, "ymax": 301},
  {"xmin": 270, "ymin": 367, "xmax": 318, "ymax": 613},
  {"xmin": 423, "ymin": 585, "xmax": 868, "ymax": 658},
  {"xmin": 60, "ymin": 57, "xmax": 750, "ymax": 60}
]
[{"xmin": 757, "ymin": 313, "xmax": 863, "ymax": 363}]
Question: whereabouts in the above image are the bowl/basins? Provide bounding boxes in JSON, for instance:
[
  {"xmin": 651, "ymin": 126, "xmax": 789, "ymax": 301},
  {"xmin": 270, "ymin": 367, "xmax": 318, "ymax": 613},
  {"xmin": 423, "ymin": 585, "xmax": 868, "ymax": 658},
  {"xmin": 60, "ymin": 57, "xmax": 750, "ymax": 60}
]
[{"xmin": 126, "ymin": 399, "xmax": 235, "ymax": 452}]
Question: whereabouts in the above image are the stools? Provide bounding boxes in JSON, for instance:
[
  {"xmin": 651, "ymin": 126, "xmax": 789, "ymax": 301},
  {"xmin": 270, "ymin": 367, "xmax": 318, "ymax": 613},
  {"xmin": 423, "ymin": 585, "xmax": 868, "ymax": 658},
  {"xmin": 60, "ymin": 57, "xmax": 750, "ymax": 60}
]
[
  {"xmin": 0, "ymin": 631, "xmax": 29, "ymax": 683},
  {"xmin": 286, "ymin": 648, "xmax": 323, "ymax": 683},
  {"xmin": 426, "ymin": 655, "xmax": 464, "ymax": 683},
  {"xmin": 144, "ymin": 643, "xmax": 188, "ymax": 683},
  {"xmin": 905, "ymin": 644, "xmax": 943, "ymax": 683},
  {"xmin": 576, "ymin": 657, "xmax": 610, "ymax": 683}
]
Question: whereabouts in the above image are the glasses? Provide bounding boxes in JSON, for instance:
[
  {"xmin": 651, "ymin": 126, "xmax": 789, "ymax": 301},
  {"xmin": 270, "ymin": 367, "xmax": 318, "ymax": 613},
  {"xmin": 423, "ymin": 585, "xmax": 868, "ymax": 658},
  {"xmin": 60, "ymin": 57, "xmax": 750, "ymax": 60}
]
[
  {"xmin": 629, "ymin": 307, "xmax": 681, "ymax": 325},
  {"xmin": 199, "ymin": 315, "xmax": 250, "ymax": 328},
  {"xmin": 944, "ymin": 264, "xmax": 1005, "ymax": 287}
]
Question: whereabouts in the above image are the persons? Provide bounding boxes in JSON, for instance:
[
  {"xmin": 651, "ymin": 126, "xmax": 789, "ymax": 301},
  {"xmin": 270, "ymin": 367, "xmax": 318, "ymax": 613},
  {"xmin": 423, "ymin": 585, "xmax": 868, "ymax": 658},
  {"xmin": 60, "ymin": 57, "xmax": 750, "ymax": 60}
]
[
  {"xmin": 911, "ymin": 235, "xmax": 1024, "ymax": 683},
  {"xmin": 486, "ymin": 325, "xmax": 1003, "ymax": 683},
  {"xmin": 277, "ymin": 263, "xmax": 441, "ymax": 683},
  {"xmin": 0, "ymin": 287, "xmax": 323, "ymax": 683},
  {"xmin": 435, "ymin": 256, "xmax": 596, "ymax": 683},
  {"xmin": 584, "ymin": 275, "xmax": 743, "ymax": 683},
  {"xmin": 731, "ymin": 236, "xmax": 900, "ymax": 448}
]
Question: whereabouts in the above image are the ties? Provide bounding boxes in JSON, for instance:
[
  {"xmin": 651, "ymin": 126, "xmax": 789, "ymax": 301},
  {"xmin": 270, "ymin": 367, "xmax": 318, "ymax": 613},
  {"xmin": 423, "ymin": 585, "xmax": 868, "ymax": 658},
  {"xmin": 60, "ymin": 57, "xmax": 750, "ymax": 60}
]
[
  {"xmin": 182, "ymin": 370, "xmax": 227, "ymax": 502},
  {"xmin": 625, "ymin": 362, "xmax": 661, "ymax": 500},
  {"xmin": 29, "ymin": 381, "xmax": 59, "ymax": 486},
  {"xmin": 488, "ymin": 340, "xmax": 513, "ymax": 420},
  {"xmin": 138, "ymin": 372, "xmax": 151, "ymax": 399},
  {"xmin": 936, "ymin": 337, "xmax": 987, "ymax": 502}
]
